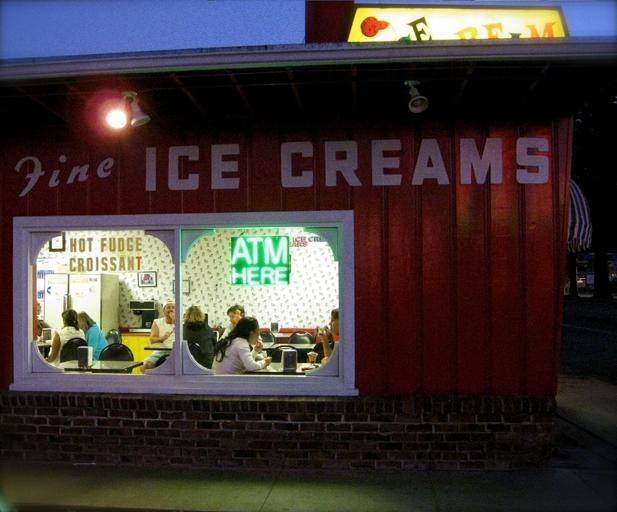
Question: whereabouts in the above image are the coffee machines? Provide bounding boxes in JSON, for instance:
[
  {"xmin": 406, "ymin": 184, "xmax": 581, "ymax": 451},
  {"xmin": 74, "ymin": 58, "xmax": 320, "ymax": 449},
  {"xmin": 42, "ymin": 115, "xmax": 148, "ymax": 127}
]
[{"xmin": 128, "ymin": 300, "xmax": 158, "ymax": 333}]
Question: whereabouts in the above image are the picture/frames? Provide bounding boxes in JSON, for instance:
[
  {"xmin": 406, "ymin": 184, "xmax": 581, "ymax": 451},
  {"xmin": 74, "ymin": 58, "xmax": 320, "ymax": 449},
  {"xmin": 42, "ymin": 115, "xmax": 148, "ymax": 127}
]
[
  {"xmin": 173, "ymin": 280, "xmax": 190, "ymax": 294},
  {"xmin": 137, "ymin": 271, "xmax": 157, "ymax": 287},
  {"xmin": 49, "ymin": 231, "xmax": 65, "ymax": 251}
]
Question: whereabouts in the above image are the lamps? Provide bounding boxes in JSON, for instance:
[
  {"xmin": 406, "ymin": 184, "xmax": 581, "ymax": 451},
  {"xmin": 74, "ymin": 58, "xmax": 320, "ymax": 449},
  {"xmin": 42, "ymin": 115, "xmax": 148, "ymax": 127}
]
[
  {"xmin": 107, "ymin": 90, "xmax": 151, "ymax": 129},
  {"xmin": 408, "ymin": 80, "xmax": 429, "ymax": 114}
]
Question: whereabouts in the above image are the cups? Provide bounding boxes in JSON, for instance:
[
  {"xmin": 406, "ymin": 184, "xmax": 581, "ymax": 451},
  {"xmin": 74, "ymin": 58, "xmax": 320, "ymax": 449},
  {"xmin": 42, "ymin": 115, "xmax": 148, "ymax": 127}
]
[{"xmin": 307, "ymin": 352, "xmax": 318, "ymax": 367}]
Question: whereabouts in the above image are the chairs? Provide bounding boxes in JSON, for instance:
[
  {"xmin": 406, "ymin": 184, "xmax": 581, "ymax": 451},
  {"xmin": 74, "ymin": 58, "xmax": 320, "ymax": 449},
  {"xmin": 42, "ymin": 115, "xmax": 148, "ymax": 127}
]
[
  {"xmin": 249, "ymin": 332, "xmax": 334, "ymax": 375},
  {"xmin": 36, "ymin": 329, "xmax": 173, "ymax": 373}
]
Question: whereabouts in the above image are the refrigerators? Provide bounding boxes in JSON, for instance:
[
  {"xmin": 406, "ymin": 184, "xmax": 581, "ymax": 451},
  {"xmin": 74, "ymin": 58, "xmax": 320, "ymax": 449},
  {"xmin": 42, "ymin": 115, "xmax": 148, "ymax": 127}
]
[{"xmin": 44, "ymin": 273, "xmax": 119, "ymax": 336}]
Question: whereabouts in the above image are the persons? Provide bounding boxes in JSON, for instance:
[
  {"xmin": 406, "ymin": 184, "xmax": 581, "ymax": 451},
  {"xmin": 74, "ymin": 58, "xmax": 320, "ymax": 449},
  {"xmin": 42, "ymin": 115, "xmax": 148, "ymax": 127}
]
[
  {"xmin": 142, "ymin": 299, "xmax": 272, "ymax": 373},
  {"xmin": 320, "ymin": 309, "xmax": 339, "ymax": 363},
  {"xmin": 37, "ymin": 303, "xmax": 108, "ymax": 363}
]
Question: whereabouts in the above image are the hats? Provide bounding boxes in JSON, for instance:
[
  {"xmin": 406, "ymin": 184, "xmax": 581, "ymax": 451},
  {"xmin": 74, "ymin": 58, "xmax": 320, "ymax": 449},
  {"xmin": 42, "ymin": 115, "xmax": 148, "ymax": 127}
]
[{"xmin": 163, "ymin": 297, "xmax": 175, "ymax": 308}]
